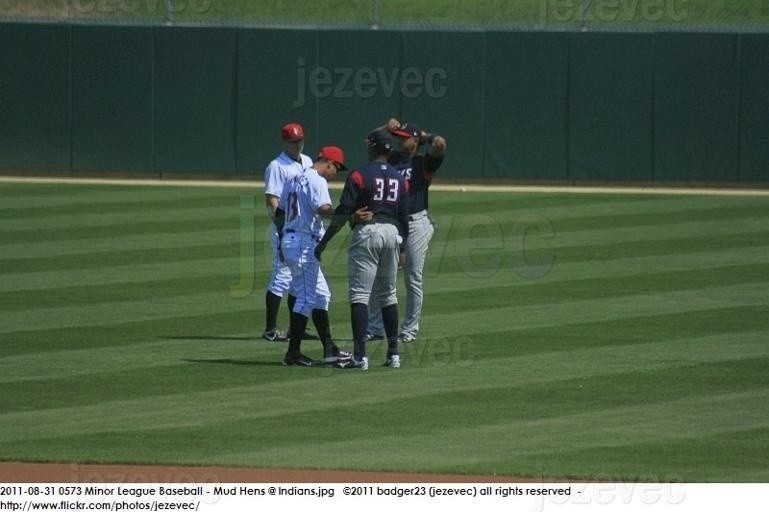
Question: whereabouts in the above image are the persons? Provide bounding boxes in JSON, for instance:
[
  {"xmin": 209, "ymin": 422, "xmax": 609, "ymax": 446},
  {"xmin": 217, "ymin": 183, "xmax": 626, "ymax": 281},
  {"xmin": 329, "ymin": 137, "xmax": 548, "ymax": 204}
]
[
  {"xmin": 262, "ymin": 124, "xmax": 318, "ymax": 344},
  {"xmin": 275, "ymin": 146, "xmax": 374, "ymax": 369},
  {"xmin": 363, "ymin": 117, "xmax": 446, "ymax": 344},
  {"xmin": 313, "ymin": 130, "xmax": 402, "ymax": 371}
]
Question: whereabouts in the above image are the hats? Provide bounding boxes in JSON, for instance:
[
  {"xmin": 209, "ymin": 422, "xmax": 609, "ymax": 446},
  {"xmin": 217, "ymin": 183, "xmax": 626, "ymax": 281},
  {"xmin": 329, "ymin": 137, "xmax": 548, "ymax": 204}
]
[
  {"xmin": 392, "ymin": 122, "xmax": 421, "ymax": 139},
  {"xmin": 281, "ymin": 122, "xmax": 305, "ymax": 142},
  {"xmin": 363, "ymin": 125, "xmax": 397, "ymax": 150},
  {"xmin": 316, "ymin": 145, "xmax": 350, "ymax": 172}
]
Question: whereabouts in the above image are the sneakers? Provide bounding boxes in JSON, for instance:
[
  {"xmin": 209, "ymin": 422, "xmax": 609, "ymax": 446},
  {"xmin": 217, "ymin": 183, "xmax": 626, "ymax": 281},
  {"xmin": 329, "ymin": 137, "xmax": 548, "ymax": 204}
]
[
  {"xmin": 365, "ymin": 332, "xmax": 384, "ymax": 342},
  {"xmin": 383, "ymin": 355, "xmax": 401, "ymax": 369},
  {"xmin": 334, "ymin": 355, "xmax": 370, "ymax": 371},
  {"xmin": 323, "ymin": 349, "xmax": 353, "ymax": 364},
  {"xmin": 282, "ymin": 352, "xmax": 323, "ymax": 368},
  {"xmin": 397, "ymin": 331, "xmax": 418, "ymax": 345},
  {"xmin": 260, "ymin": 327, "xmax": 291, "ymax": 343}
]
